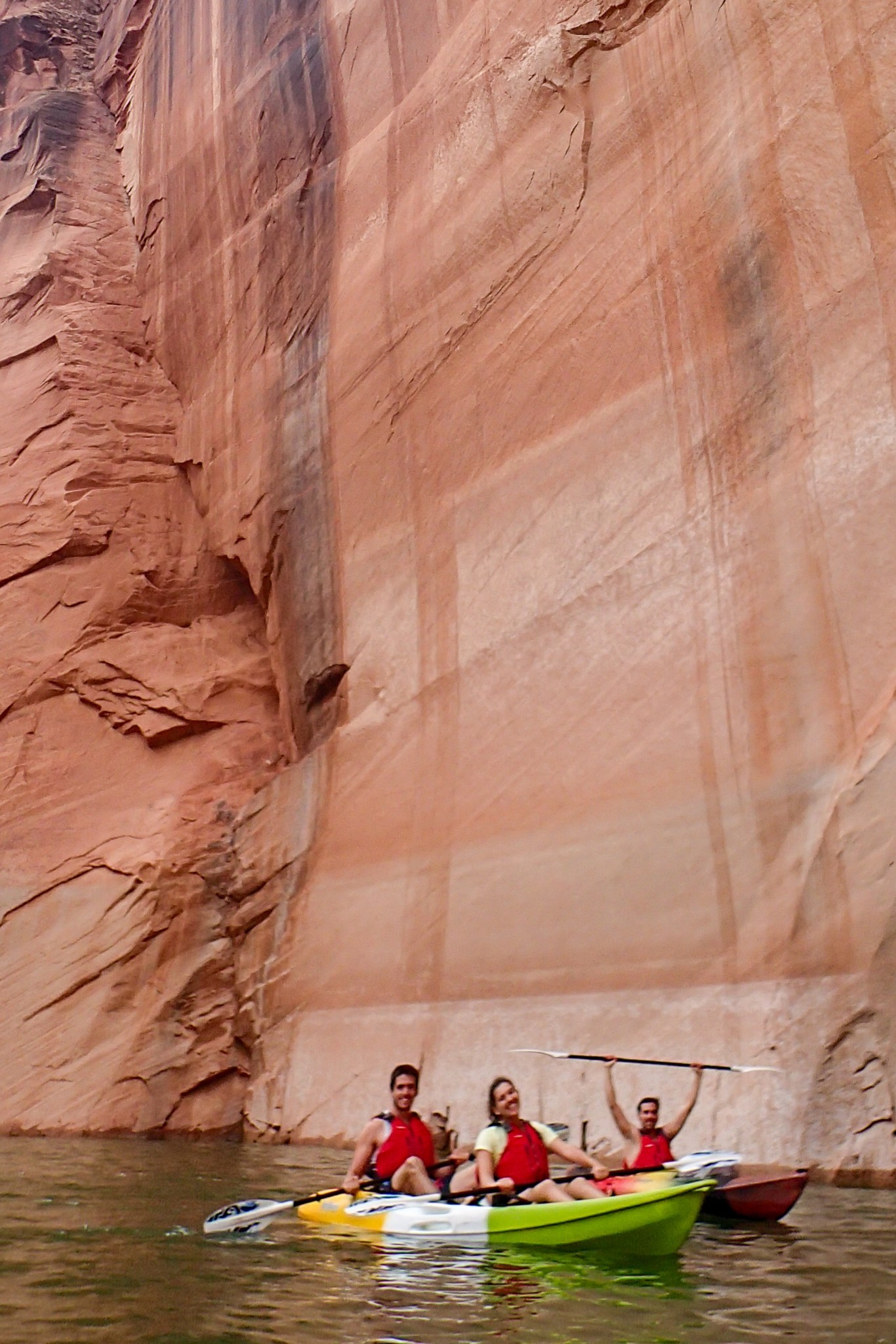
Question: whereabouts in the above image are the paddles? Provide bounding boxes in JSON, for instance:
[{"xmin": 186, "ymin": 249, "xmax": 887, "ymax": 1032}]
[
  {"xmin": 510, "ymin": 1048, "xmax": 786, "ymax": 1077},
  {"xmin": 344, "ymin": 1149, "xmax": 742, "ymax": 1218},
  {"xmin": 203, "ymin": 1122, "xmax": 572, "ymax": 1234}
]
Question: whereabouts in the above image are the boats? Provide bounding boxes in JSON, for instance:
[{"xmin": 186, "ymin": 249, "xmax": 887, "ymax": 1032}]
[
  {"xmin": 549, "ymin": 1160, "xmax": 811, "ymax": 1224},
  {"xmin": 295, "ymin": 1176, "xmax": 721, "ymax": 1258}
]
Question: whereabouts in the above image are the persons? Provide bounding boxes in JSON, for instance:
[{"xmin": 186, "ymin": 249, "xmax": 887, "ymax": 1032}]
[
  {"xmin": 341, "ymin": 1064, "xmax": 480, "ymax": 1205},
  {"xmin": 603, "ymin": 1056, "xmax": 703, "ymax": 1172},
  {"xmin": 473, "ymin": 1076, "xmax": 612, "ymax": 1207},
  {"xmin": 428, "ymin": 1112, "xmax": 459, "ymax": 1159}
]
